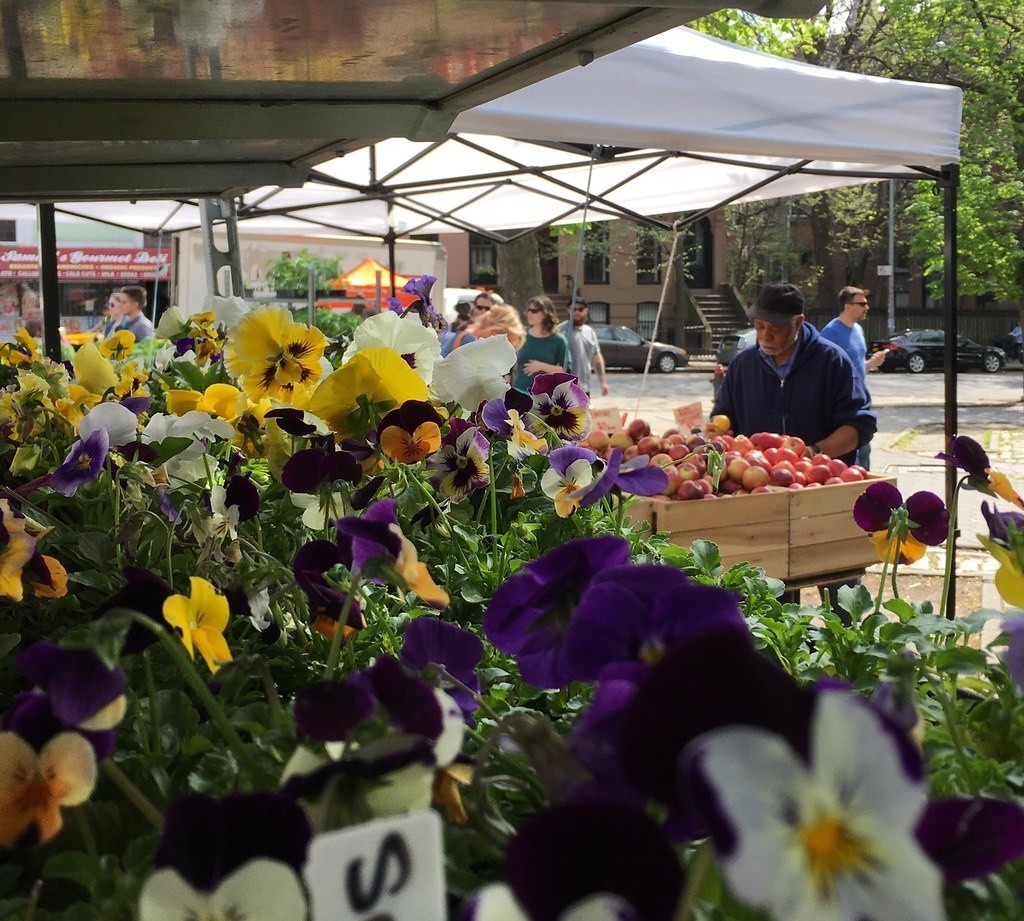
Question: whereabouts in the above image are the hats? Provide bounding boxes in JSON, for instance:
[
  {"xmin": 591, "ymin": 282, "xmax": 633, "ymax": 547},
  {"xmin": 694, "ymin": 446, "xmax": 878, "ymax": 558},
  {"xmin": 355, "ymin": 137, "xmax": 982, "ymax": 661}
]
[
  {"xmin": 746, "ymin": 282, "xmax": 805, "ymax": 324},
  {"xmin": 455, "ymin": 300, "xmax": 470, "ymax": 313},
  {"xmin": 567, "ymin": 297, "xmax": 588, "ymax": 307}
]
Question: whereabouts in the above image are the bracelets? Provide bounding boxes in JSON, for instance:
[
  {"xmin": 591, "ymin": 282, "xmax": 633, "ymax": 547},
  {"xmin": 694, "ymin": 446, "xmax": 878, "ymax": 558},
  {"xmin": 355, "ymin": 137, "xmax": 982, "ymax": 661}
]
[{"xmin": 811, "ymin": 443, "xmax": 822, "ymax": 455}]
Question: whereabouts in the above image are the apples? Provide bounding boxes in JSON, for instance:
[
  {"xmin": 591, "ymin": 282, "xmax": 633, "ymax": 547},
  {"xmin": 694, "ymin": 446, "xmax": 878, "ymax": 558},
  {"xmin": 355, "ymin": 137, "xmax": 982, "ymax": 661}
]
[{"xmin": 576, "ymin": 415, "xmax": 868, "ymax": 503}]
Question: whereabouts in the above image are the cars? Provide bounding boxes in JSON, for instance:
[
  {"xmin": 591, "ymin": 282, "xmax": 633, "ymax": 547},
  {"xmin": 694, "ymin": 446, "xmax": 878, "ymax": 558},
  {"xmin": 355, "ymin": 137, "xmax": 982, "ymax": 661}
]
[
  {"xmin": 717, "ymin": 328, "xmax": 757, "ymax": 365},
  {"xmin": 556, "ymin": 320, "xmax": 689, "ymax": 373},
  {"xmin": 866, "ymin": 328, "xmax": 1007, "ymax": 375}
]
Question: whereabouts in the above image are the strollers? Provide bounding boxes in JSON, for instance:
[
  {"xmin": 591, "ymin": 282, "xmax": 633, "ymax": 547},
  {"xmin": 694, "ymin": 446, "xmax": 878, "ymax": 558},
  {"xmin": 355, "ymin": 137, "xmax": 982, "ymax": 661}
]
[{"xmin": 990, "ymin": 332, "xmax": 1017, "ymax": 360}]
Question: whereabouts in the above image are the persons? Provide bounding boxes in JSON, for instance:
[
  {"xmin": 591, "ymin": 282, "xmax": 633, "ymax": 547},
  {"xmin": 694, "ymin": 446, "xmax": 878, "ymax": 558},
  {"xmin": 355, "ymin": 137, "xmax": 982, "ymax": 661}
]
[
  {"xmin": 437, "ymin": 291, "xmax": 527, "ymax": 358},
  {"xmin": 119, "ymin": 286, "xmax": 154, "ymax": 343},
  {"xmin": 820, "ymin": 286, "xmax": 886, "ymax": 474},
  {"xmin": 1008, "ymin": 320, "xmax": 1022, "ymax": 361},
  {"xmin": 25, "ymin": 320, "xmax": 44, "ymax": 356},
  {"xmin": 102, "ymin": 292, "xmax": 129, "ymax": 341},
  {"xmin": 88, "ymin": 303, "xmax": 112, "ymax": 333},
  {"xmin": 555, "ymin": 296, "xmax": 608, "ymax": 398},
  {"xmin": 505, "ymin": 294, "xmax": 567, "ymax": 390},
  {"xmin": 707, "ymin": 282, "xmax": 878, "ymax": 652}
]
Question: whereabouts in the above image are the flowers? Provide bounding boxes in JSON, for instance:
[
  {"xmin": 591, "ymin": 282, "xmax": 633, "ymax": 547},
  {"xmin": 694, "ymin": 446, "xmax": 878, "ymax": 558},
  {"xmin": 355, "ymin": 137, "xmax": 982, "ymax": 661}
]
[{"xmin": 0, "ymin": 273, "xmax": 1024, "ymax": 921}]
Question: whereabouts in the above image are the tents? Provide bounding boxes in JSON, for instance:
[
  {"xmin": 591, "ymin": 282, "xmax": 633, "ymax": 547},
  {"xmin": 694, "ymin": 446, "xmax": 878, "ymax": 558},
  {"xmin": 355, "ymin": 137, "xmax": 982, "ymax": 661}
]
[{"xmin": 0, "ymin": 26, "xmax": 965, "ymax": 621}]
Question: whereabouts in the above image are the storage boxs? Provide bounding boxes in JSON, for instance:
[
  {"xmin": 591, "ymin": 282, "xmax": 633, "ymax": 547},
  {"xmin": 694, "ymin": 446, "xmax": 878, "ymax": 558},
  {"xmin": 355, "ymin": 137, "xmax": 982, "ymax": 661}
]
[
  {"xmin": 769, "ymin": 472, "xmax": 896, "ymax": 578},
  {"xmin": 621, "ymin": 489, "xmax": 790, "ymax": 579}
]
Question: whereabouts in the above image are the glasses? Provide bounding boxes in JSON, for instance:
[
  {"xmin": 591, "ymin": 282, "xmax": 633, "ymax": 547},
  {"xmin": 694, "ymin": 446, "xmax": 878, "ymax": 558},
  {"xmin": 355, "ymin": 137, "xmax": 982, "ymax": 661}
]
[
  {"xmin": 477, "ymin": 305, "xmax": 491, "ymax": 311},
  {"xmin": 851, "ymin": 302, "xmax": 869, "ymax": 307},
  {"xmin": 527, "ymin": 309, "xmax": 542, "ymax": 313},
  {"xmin": 574, "ymin": 307, "xmax": 586, "ymax": 312}
]
